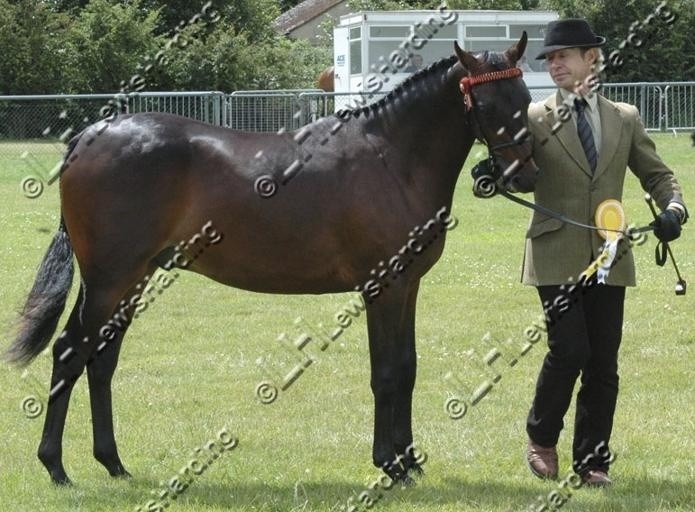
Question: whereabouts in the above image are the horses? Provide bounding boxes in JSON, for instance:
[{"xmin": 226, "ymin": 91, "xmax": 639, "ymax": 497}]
[{"xmin": 4, "ymin": 28, "xmax": 541, "ymax": 488}]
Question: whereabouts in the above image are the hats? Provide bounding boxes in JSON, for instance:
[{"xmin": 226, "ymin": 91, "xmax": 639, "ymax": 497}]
[{"xmin": 534, "ymin": 18, "xmax": 608, "ymax": 60}]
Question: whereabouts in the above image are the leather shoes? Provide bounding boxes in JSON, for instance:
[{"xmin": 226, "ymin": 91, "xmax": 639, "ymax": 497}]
[
  {"xmin": 582, "ymin": 470, "xmax": 613, "ymax": 487},
  {"xmin": 526, "ymin": 438, "xmax": 560, "ymax": 481}
]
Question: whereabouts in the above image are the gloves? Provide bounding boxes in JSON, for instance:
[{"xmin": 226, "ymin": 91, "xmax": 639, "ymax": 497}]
[
  {"xmin": 471, "ymin": 158, "xmax": 494, "ymax": 180},
  {"xmin": 648, "ymin": 210, "xmax": 683, "ymax": 243}
]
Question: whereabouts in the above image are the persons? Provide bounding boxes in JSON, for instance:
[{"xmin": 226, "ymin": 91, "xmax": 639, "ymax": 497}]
[
  {"xmin": 527, "ymin": 20, "xmax": 686, "ymax": 489},
  {"xmin": 402, "ymin": 53, "xmax": 424, "ymax": 72}
]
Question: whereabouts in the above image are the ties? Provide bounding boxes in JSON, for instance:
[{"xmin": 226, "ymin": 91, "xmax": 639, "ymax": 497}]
[{"xmin": 574, "ymin": 97, "xmax": 598, "ymax": 175}]
